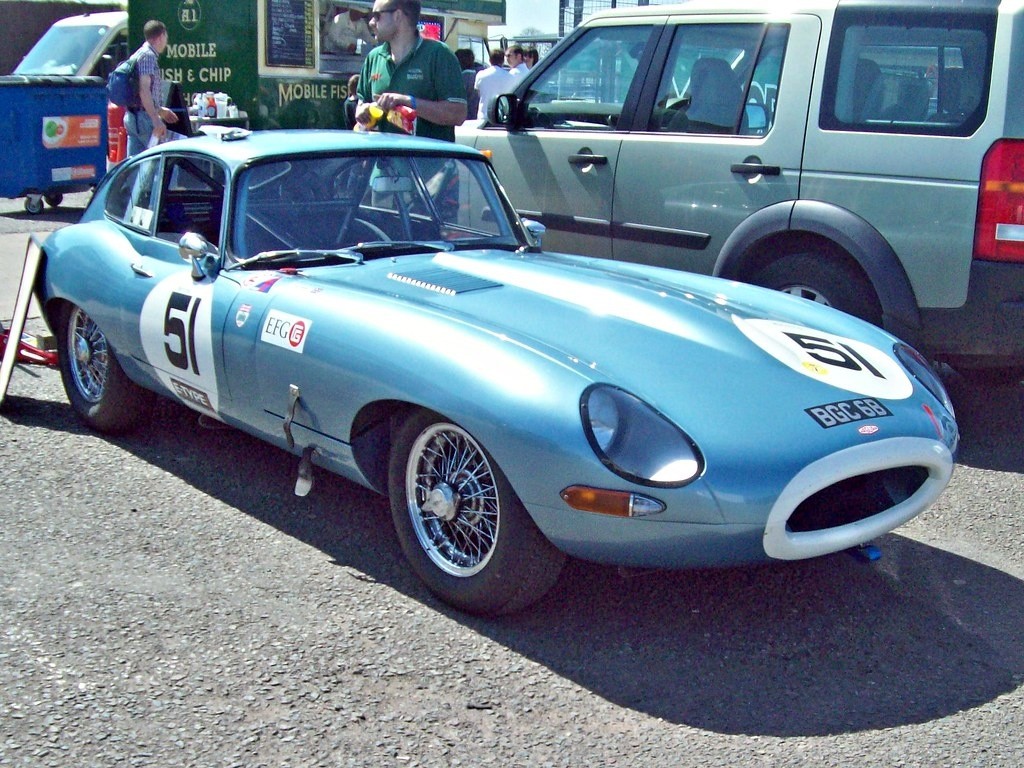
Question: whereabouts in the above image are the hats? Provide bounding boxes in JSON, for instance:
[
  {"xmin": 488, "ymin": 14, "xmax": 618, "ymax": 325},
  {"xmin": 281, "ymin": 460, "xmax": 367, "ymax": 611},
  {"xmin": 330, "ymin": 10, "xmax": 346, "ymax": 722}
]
[{"xmin": 349, "ymin": 4, "xmax": 370, "ymax": 13}]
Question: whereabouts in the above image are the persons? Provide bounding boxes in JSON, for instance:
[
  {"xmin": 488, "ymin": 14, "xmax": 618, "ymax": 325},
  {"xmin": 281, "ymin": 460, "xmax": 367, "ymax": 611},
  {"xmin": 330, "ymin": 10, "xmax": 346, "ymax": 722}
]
[
  {"xmin": 455, "ymin": 46, "xmax": 538, "ymax": 120},
  {"xmin": 344, "ymin": 75, "xmax": 359, "ymax": 131},
  {"xmin": 355, "ymin": 0, "xmax": 467, "ymax": 225},
  {"xmin": 323, "ymin": 4, "xmax": 376, "ymax": 53},
  {"xmin": 124, "ymin": 19, "xmax": 179, "ymax": 195}
]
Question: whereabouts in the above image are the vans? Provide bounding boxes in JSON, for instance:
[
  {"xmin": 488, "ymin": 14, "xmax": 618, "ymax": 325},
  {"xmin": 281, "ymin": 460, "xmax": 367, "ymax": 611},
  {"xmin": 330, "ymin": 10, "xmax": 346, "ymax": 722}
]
[
  {"xmin": 8, "ymin": 9, "xmax": 128, "ymax": 78},
  {"xmin": 444, "ymin": 0, "xmax": 1024, "ymax": 378}
]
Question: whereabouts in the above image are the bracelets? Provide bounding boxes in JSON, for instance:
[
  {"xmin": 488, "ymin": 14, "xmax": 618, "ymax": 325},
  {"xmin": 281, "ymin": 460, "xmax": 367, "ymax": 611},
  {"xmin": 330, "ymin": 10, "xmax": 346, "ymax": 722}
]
[{"xmin": 409, "ymin": 95, "xmax": 416, "ymax": 110}]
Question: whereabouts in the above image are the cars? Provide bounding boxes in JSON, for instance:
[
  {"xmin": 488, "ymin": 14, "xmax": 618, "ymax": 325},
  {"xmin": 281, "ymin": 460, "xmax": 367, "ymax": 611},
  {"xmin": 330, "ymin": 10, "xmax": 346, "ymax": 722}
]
[{"xmin": 31, "ymin": 126, "xmax": 958, "ymax": 620}]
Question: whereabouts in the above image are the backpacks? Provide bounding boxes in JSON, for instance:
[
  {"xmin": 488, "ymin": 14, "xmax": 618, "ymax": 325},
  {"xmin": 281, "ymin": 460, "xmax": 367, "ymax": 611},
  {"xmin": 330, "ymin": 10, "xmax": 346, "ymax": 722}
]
[{"xmin": 106, "ymin": 48, "xmax": 147, "ymax": 107}]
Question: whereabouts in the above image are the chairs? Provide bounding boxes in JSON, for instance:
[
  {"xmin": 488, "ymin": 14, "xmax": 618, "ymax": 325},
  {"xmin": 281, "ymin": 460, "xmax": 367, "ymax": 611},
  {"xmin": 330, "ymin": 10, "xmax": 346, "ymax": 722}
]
[
  {"xmin": 833, "ymin": 58, "xmax": 964, "ymax": 125},
  {"xmin": 667, "ymin": 58, "xmax": 750, "ymax": 136}
]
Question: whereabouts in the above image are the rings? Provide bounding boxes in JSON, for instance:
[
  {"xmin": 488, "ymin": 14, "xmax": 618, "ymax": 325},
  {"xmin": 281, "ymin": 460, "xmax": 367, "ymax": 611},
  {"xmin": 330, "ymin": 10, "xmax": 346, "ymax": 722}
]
[{"xmin": 160, "ymin": 130, "xmax": 163, "ymax": 132}]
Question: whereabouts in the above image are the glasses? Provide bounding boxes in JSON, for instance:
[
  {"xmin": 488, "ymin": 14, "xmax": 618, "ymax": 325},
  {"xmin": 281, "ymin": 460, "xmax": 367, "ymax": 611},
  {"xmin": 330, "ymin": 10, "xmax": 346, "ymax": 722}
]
[
  {"xmin": 506, "ymin": 54, "xmax": 517, "ymax": 58},
  {"xmin": 366, "ymin": 9, "xmax": 397, "ymax": 21}
]
